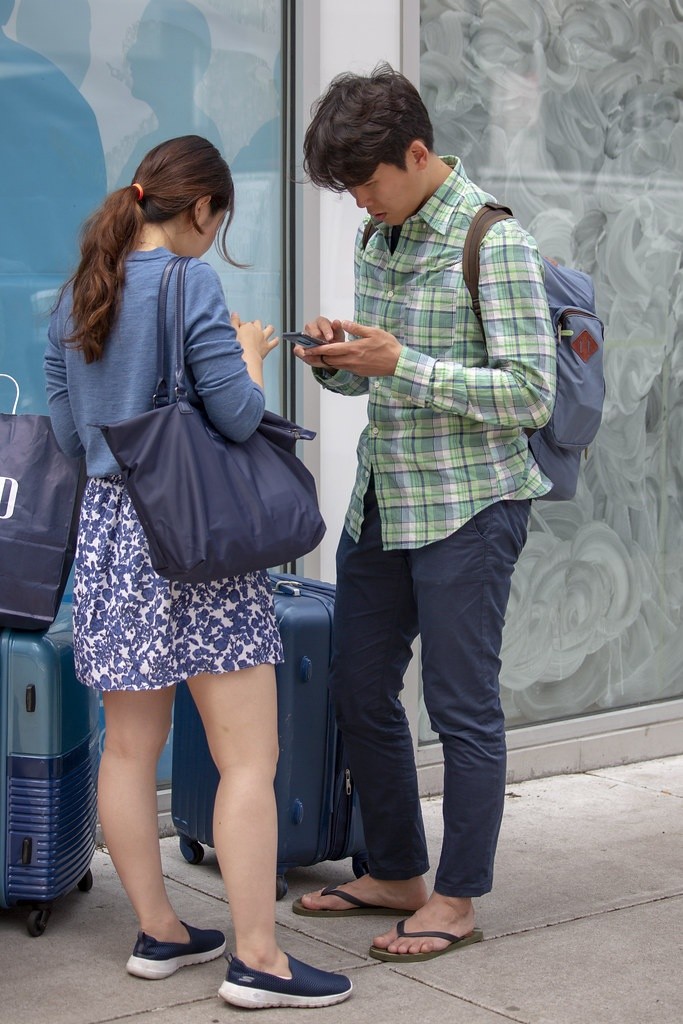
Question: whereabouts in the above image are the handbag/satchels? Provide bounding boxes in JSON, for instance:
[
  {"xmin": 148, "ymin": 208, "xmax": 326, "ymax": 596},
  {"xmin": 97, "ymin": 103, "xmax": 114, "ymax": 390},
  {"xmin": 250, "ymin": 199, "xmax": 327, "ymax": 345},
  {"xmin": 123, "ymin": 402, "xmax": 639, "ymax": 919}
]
[
  {"xmin": 0, "ymin": 374, "xmax": 86, "ymax": 634},
  {"xmin": 87, "ymin": 256, "xmax": 326, "ymax": 582}
]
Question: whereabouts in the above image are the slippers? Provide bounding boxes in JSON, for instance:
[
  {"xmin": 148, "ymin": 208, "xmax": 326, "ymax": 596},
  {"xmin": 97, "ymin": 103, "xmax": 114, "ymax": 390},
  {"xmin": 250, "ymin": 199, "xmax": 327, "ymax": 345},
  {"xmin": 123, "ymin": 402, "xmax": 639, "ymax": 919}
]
[
  {"xmin": 368, "ymin": 918, "xmax": 483, "ymax": 963},
  {"xmin": 291, "ymin": 880, "xmax": 417, "ymax": 918}
]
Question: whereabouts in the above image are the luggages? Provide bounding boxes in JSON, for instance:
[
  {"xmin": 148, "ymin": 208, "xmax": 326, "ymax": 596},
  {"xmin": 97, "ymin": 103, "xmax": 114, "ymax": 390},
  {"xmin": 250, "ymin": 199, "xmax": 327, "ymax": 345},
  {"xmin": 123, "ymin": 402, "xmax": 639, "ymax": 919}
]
[
  {"xmin": 169, "ymin": 572, "xmax": 370, "ymax": 900},
  {"xmin": 0, "ymin": 601, "xmax": 98, "ymax": 938}
]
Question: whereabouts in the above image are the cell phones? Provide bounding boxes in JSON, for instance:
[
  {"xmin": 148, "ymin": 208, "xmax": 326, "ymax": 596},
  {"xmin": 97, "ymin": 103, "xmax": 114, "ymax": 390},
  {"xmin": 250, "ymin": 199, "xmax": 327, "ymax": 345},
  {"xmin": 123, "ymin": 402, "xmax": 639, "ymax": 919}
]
[{"xmin": 282, "ymin": 332, "xmax": 327, "ymax": 348}]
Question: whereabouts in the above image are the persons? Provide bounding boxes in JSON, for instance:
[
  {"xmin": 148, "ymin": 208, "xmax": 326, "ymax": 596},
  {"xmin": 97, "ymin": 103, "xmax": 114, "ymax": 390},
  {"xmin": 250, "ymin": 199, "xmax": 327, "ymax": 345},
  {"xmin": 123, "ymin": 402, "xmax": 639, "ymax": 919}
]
[
  {"xmin": 292, "ymin": 61, "xmax": 561, "ymax": 960},
  {"xmin": 43, "ymin": 134, "xmax": 352, "ymax": 1009}
]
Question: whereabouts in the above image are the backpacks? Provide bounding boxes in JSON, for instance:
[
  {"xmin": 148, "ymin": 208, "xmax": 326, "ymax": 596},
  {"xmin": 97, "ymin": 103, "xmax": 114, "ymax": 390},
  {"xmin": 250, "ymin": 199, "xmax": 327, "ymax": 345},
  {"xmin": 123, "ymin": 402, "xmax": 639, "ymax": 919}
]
[{"xmin": 460, "ymin": 203, "xmax": 606, "ymax": 503}]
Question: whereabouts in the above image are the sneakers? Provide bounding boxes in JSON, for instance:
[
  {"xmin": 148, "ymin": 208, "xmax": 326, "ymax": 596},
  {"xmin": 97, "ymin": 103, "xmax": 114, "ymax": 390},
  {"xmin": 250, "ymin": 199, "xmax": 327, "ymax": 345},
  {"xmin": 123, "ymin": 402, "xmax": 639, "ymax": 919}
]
[
  {"xmin": 217, "ymin": 951, "xmax": 353, "ymax": 1009},
  {"xmin": 126, "ymin": 920, "xmax": 226, "ymax": 980}
]
